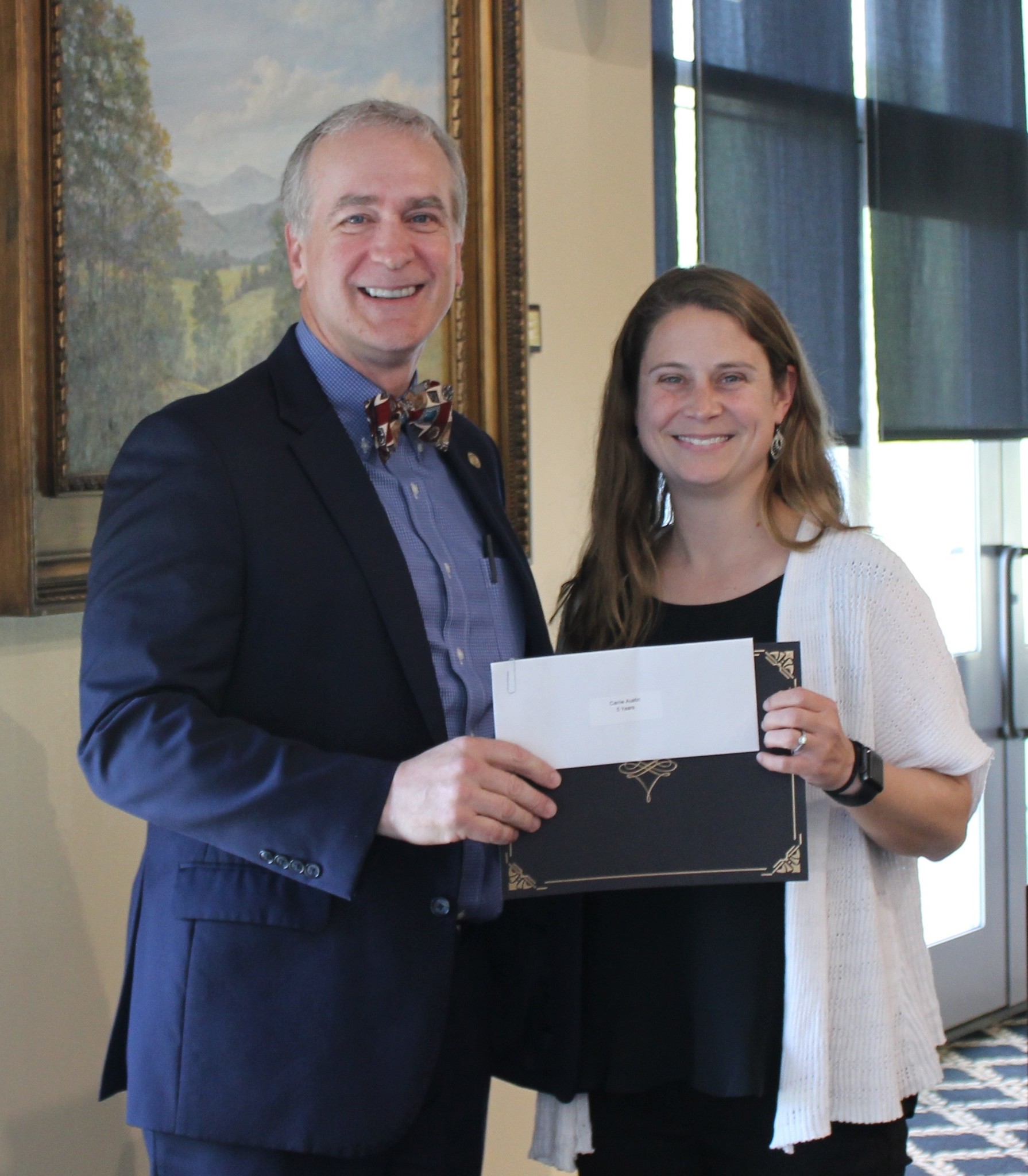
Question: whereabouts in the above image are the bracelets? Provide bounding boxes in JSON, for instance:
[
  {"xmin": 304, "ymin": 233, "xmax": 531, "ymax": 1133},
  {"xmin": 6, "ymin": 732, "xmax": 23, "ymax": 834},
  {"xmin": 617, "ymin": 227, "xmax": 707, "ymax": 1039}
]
[{"xmin": 822, "ymin": 740, "xmax": 863, "ymax": 795}]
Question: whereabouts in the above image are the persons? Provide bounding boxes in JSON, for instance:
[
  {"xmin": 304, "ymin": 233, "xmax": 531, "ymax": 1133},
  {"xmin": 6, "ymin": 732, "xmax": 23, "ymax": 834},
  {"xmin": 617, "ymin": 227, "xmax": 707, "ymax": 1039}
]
[
  {"xmin": 82, "ymin": 99, "xmax": 549, "ymax": 1176},
  {"xmin": 525, "ymin": 263, "xmax": 999, "ymax": 1176}
]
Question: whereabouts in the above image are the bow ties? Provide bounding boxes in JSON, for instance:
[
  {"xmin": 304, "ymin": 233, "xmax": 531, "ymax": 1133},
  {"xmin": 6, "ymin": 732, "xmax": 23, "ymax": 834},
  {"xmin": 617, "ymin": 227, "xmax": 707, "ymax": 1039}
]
[{"xmin": 362, "ymin": 379, "xmax": 454, "ymax": 462}]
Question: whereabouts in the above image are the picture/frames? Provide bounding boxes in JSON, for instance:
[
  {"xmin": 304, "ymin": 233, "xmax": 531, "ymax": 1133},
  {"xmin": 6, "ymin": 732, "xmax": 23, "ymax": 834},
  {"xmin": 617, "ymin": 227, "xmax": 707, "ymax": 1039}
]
[{"xmin": 0, "ymin": 0, "xmax": 536, "ymax": 624}]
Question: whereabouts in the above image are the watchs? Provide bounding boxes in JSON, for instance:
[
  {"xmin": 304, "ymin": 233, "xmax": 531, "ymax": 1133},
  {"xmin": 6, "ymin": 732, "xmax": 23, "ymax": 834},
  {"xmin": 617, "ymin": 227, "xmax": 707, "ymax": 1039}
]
[{"xmin": 824, "ymin": 739, "xmax": 885, "ymax": 807}]
